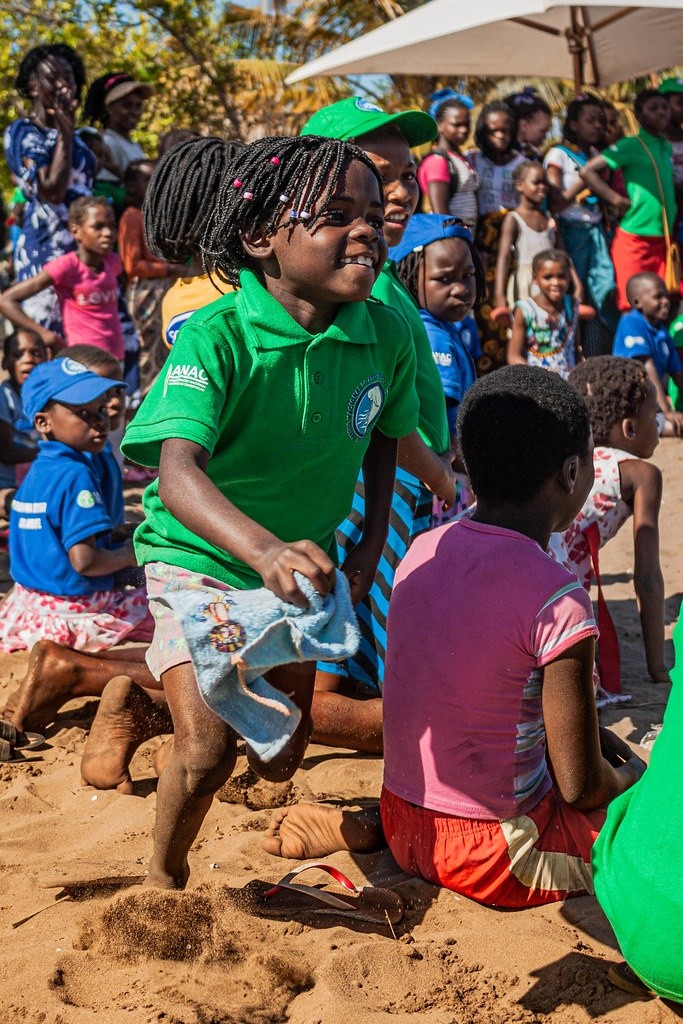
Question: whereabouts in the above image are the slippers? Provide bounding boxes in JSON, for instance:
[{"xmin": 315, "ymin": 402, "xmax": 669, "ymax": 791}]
[{"xmin": 239, "ymin": 862, "xmax": 404, "ymax": 925}]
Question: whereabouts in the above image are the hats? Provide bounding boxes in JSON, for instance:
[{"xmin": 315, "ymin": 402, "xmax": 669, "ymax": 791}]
[
  {"xmin": 12, "ymin": 41, "xmax": 88, "ymax": 102},
  {"xmin": 83, "ymin": 71, "xmax": 154, "ymax": 126},
  {"xmin": 429, "ymin": 86, "xmax": 474, "ymax": 121},
  {"xmin": 387, "ymin": 213, "xmax": 473, "ymax": 265},
  {"xmin": 659, "ymin": 76, "xmax": 683, "ymax": 95},
  {"xmin": 299, "ymin": 96, "xmax": 439, "ymax": 148},
  {"xmin": 20, "ymin": 357, "xmax": 129, "ymax": 428}
]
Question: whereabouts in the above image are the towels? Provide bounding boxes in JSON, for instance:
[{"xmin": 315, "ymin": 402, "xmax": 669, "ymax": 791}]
[{"xmin": 141, "ymin": 567, "xmax": 363, "ymax": 765}]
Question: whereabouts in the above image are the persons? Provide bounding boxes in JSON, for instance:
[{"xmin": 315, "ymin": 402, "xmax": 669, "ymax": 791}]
[
  {"xmin": 588, "ymin": 599, "xmax": 682, "ymax": 1011},
  {"xmin": 0, "ymin": 72, "xmax": 683, "ymax": 792},
  {"xmin": 507, "ymin": 249, "xmax": 578, "ymax": 381},
  {"xmin": 610, "ymin": 272, "xmax": 683, "ymax": 437},
  {"xmin": 84, "ymin": 67, "xmax": 155, "ymax": 295},
  {"xmin": 448, "ymin": 353, "xmax": 665, "ymax": 703},
  {"xmin": 264, "ymin": 365, "xmax": 641, "ymax": 911},
  {"xmin": 120, "ymin": 137, "xmax": 420, "ymax": 895},
  {"xmin": 6, "ymin": 43, "xmax": 140, "ymax": 413},
  {"xmin": 667, "ymin": 280, "xmax": 683, "ymax": 412}
]
